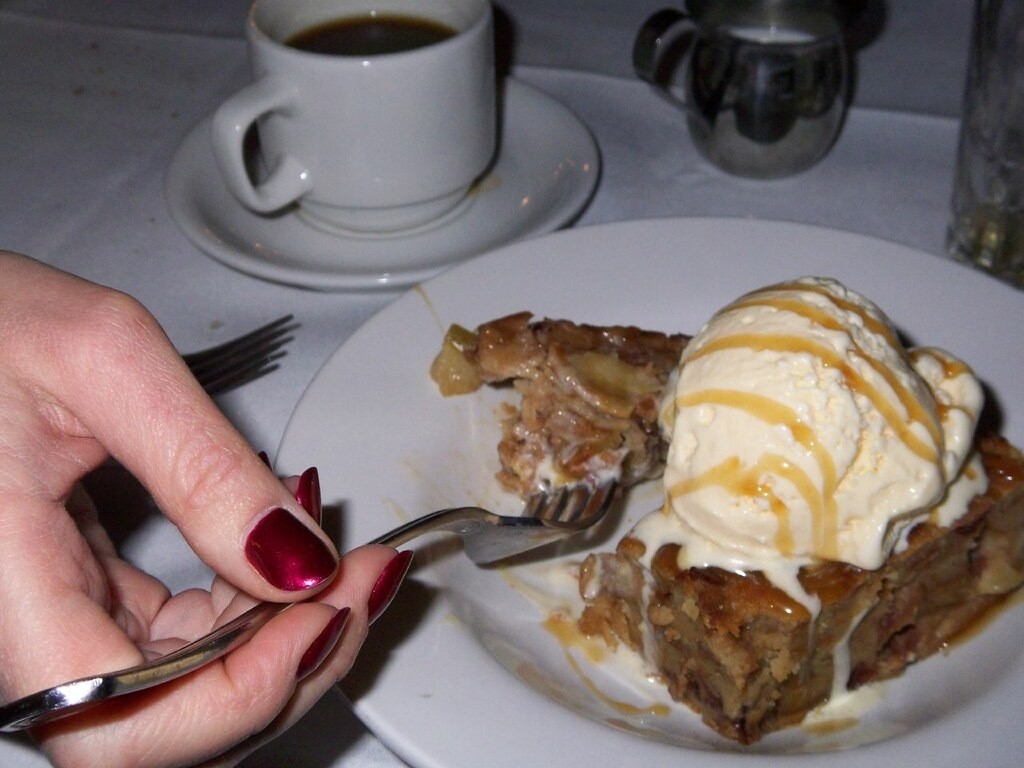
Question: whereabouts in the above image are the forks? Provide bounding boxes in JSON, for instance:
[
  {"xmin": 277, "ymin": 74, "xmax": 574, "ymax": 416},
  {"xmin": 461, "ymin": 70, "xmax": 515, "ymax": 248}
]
[
  {"xmin": 177, "ymin": 311, "xmax": 294, "ymax": 396},
  {"xmin": 0, "ymin": 475, "xmax": 618, "ymax": 735}
]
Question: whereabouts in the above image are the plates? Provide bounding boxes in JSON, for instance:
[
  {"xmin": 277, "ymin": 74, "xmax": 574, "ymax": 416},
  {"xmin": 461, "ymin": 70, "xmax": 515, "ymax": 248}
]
[
  {"xmin": 268, "ymin": 215, "xmax": 1024, "ymax": 767},
  {"xmin": 162, "ymin": 74, "xmax": 602, "ymax": 292}
]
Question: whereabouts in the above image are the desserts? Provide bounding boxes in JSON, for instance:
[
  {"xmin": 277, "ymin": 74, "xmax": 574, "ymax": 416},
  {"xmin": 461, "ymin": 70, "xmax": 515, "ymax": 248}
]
[{"xmin": 432, "ymin": 273, "xmax": 1023, "ymax": 749}]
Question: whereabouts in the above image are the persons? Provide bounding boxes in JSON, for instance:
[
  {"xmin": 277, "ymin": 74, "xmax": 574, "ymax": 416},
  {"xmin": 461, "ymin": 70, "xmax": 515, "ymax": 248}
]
[{"xmin": 0, "ymin": 249, "xmax": 415, "ymax": 768}]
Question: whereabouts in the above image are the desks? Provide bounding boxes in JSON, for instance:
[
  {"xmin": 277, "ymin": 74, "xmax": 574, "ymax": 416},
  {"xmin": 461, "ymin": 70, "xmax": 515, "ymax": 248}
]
[{"xmin": 0, "ymin": 1, "xmax": 972, "ymax": 768}]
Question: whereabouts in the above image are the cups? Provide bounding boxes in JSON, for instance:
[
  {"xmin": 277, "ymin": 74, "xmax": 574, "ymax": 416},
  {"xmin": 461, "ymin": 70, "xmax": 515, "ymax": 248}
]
[
  {"xmin": 631, "ymin": 0, "xmax": 854, "ymax": 181},
  {"xmin": 209, "ymin": 1, "xmax": 497, "ymax": 234},
  {"xmin": 944, "ymin": 1, "xmax": 1024, "ymax": 291}
]
